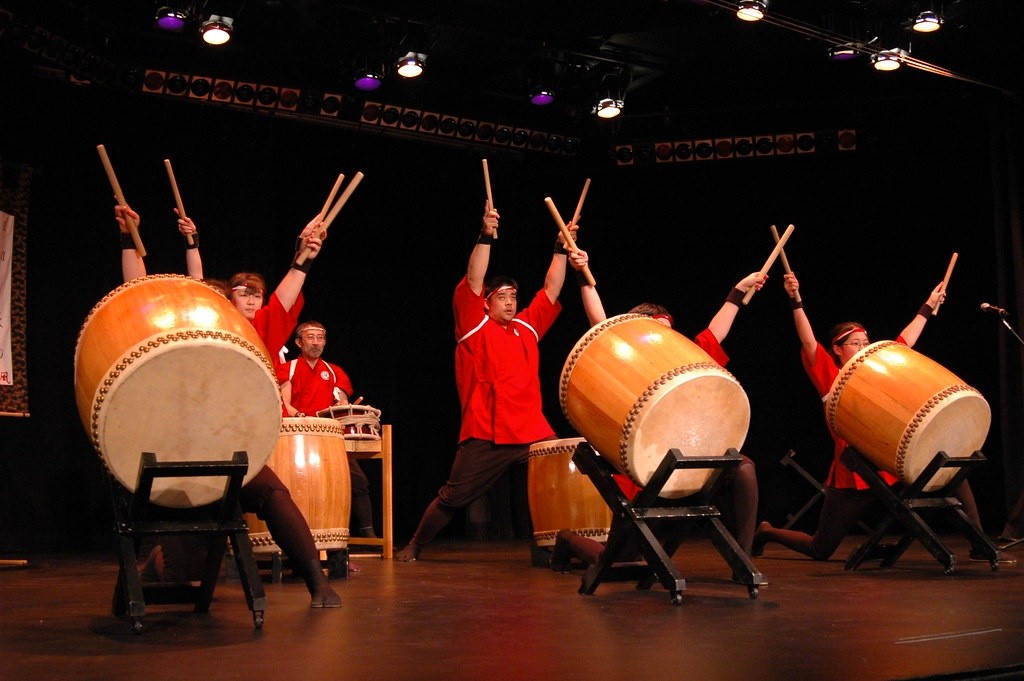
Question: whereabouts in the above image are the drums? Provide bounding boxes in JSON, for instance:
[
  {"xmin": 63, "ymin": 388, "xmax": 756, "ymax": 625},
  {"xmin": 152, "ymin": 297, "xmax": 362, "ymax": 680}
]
[
  {"xmin": 527, "ymin": 437, "xmax": 613, "ymax": 548},
  {"xmin": 558, "ymin": 312, "xmax": 753, "ymax": 500},
  {"xmin": 315, "ymin": 404, "xmax": 382, "ymax": 440},
  {"xmin": 827, "ymin": 340, "xmax": 992, "ymax": 495},
  {"xmin": 72, "ymin": 272, "xmax": 286, "ymax": 509},
  {"xmin": 225, "ymin": 414, "xmax": 353, "ymax": 556}
]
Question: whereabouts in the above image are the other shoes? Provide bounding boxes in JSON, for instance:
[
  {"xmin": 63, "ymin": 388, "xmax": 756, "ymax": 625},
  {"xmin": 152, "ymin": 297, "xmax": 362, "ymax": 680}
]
[
  {"xmin": 968, "ymin": 549, "xmax": 1018, "ymax": 563},
  {"xmin": 362, "ymin": 546, "xmax": 397, "ymax": 554}
]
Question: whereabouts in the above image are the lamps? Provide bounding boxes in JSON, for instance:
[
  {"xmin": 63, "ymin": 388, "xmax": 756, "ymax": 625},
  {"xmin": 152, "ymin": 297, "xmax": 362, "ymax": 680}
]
[
  {"xmin": 154, "ymin": 0, "xmax": 199, "ymax": 34},
  {"xmin": 349, "ymin": 45, "xmax": 386, "ymax": 89},
  {"xmin": 911, "ymin": 0, "xmax": 943, "ymax": 33},
  {"xmin": 736, "ymin": 0, "xmax": 770, "ymax": 21},
  {"xmin": 394, "ymin": 40, "xmax": 427, "ymax": 78},
  {"xmin": 870, "ymin": 17, "xmax": 911, "ymax": 71},
  {"xmin": 527, "ymin": 69, "xmax": 555, "ymax": 106},
  {"xmin": 197, "ymin": 7, "xmax": 234, "ymax": 46},
  {"xmin": 590, "ymin": 66, "xmax": 626, "ymax": 119},
  {"xmin": 821, "ymin": 12, "xmax": 861, "ymax": 59}
]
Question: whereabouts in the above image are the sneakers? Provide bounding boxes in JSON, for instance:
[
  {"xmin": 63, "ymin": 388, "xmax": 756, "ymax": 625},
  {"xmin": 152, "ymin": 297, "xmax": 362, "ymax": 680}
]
[{"xmin": 310, "ymin": 591, "xmax": 341, "ymax": 608}]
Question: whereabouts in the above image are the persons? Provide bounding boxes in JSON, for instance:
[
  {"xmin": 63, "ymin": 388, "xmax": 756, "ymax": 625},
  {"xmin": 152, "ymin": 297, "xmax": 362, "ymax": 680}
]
[
  {"xmin": 752, "ymin": 271, "xmax": 952, "ymax": 558},
  {"xmin": 113, "ymin": 207, "xmax": 357, "ymax": 607},
  {"xmin": 391, "ymin": 197, "xmax": 581, "ymax": 564},
  {"xmin": 558, "ymin": 245, "xmax": 769, "ymax": 587}
]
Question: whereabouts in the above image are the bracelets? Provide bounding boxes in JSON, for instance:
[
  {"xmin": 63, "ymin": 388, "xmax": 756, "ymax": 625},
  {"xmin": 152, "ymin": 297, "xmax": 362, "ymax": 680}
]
[
  {"xmin": 120, "ymin": 231, "xmax": 138, "ymax": 250},
  {"xmin": 916, "ymin": 304, "xmax": 934, "ymax": 321},
  {"xmin": 576, "ymin": 269, "xmax": 591, "ymax": 287},
  {"xmin": 295, "ymin": 235, "xmax": 303, "ymax": 250},
  {"xmin": 553, "ymin": 241, "xmax": 569, "ymax": 256},
  {"xmin": 291, "ymin": 251, "xmax": 312, "ymax": 273},
  {"xmin": 723, "ymin": 287, "xmax": 748, "ymax": 309},
  {"xmin": 789, "ymin": 296, "xmax": 804, "ymax": 311},
  {"xmin": 184, "ymin": 232, "xmax": 200, "ymax": 250},
  {"xmin": 475, "ymin": 231, "xmax": 492, "ymax": 245}
]
